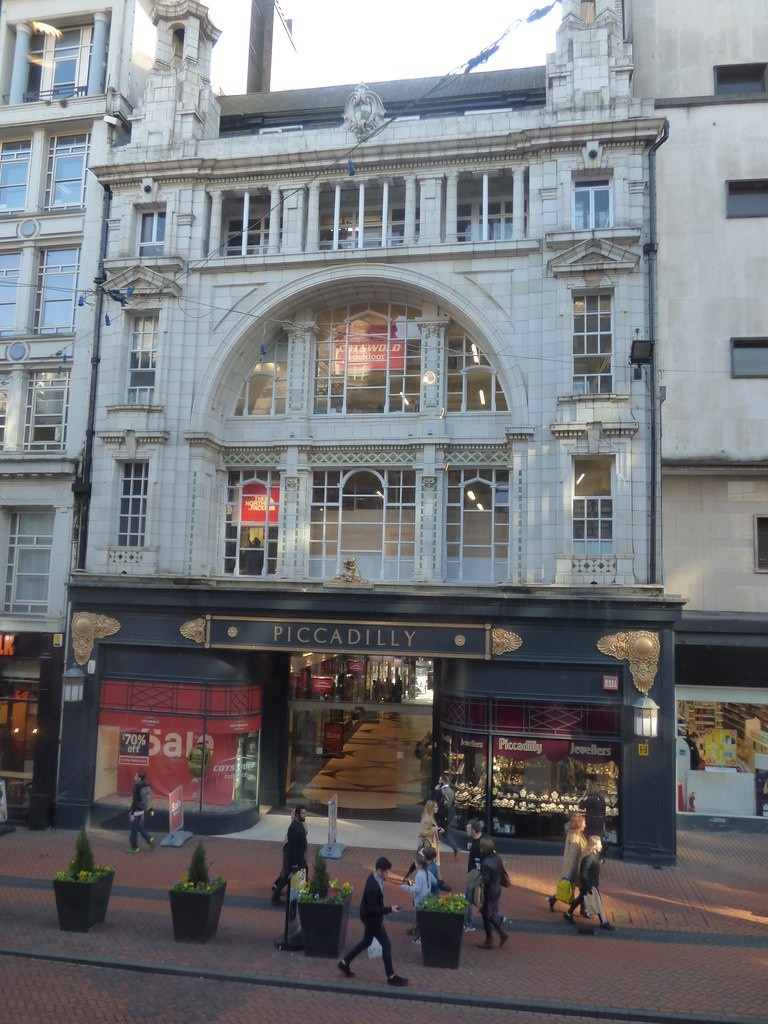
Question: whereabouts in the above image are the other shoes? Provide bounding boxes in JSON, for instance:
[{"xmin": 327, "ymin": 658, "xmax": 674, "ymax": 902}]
[
  {"xmin": 412, "ymin": 936, "xmax": 421, "ymax": 945},
  {"xmin": 403, "ymin": 877, "xmax": 413, "ymax": 885},
  {"xmin": 453, "ymin": 848, "xmax": 461, "ymax": 859},
  {"xmin": 125, "ymin": 847, "xmax": 140, "ymax": 854},
  {"xmin": 387, "ymin": 974, "xmax": 408, "ymax": 986},
  {"xmin": 338, "ymin": 961, "xmax": 355, "ymax": 977},
  {"xmin": 500, "ymin": 916, "xmax": 506, "ymax": 924},
  {"xmin": 149, "ymin": 838, "xmax": 156, "ymax": 850},
  {"xmin": 580, "ymin": 910, "xmax": 593, "ymax": 919},
  {"xmin": 600, "ymin": 921, "xmax": 615, "ymax": 930},
  {"xmin": 548, "ymin": 896, "xmax": 556, "ymax": 912},
  {"xmin": 272, "ymin": 885, "xmax": 277, "ymax": 891},
  {"xmin": 271, "ymin": 899, "xmax": 285, "ymax": 905},
  {"xmin": 463, "ymin": 924, "xmax": 475, "ymax": 932},
  {"xmin": 564, "ymin": 912, "xmax": 576, "ymax": 923}
]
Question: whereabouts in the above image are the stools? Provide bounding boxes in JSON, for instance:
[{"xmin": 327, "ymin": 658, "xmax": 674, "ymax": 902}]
[{"xmin": 6, "ymin": 782, "xmax": 23, "ymax": 805}]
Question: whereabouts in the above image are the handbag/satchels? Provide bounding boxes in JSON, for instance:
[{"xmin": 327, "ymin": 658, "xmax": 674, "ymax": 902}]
[
  {"xmin": 439, "ymin": 880, "xmax": 451, "ymax": 892},
  {"xmin": 555, "ymin": 877, "xmax": 575, "ymax": 904},
  {"xmin": 500, "ymin": 870, "xmax": 511, "ymax": 887}
]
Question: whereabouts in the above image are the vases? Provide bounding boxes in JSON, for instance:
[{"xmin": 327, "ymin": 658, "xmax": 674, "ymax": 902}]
[
  {"xmin": 416, "ymin": 911, "xmax": 465, "ymax": 969},
  {"xmin": 54, "ymin": 872, "xmax": 115, "ymax": 933},
  {"xmin": 298, "ymin": 894, "xmax": 352, "ymax": 959},
  {"xmin": 169, "ymin": 881, "xmax": 227, "ymax": 944}
]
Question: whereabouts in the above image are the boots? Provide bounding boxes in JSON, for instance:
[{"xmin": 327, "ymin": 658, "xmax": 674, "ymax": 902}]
[
  {"xmin": 477, "ymin": 936, "xmax": 494, "ymax": 949},
  {"xmin": 498, "ymin": 928, "xmax": 508, "ymax": 946}
]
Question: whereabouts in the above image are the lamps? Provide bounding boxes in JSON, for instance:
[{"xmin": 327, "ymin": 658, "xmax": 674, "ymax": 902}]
[
  {"xmin": 632, "ymin": 691, "xmax": 660, "ymax": 742},
  {"xmin": 62, "ymin": 663, "xmax": 86, "ymax": 702},
  {"xmin": 629, "ymin": 340, "xmax": 655, "ymax": 380}
]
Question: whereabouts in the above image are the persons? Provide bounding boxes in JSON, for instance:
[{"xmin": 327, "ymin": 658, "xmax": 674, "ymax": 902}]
[
  {"xmin": 686, "ymin": 732, "xmax": 703, "ymax": 770},
  {"xmin": 547, "ymin": 815, "xmax": 593, "ymax": 918},
  {"xmin": 463, "ymin": 819, "xmax": 510, "ymax": 947},
  {"xmin": 562, "ymin": 835, "xmax": 615, "ymax": 931},
  {"xmin": 340, "ymin": 557, "xmax": 361, "ymax": 582},
  {"xmin": 579, "ymin": 774, "xmax": 607, "ymax": 863},
  {"xmin": 401, "ymin": 845, "xmax": 440, "ymax": 946},
  {"xmin": 271, "ymin": 805, "xmax": 308, "ymax": 905},
  {"xmin": 125, "ymin": 771, "xmax": 156, "ymax": 853},
  {"xmin": 431, "ymin": 775, "xmax": 460, "ymax": 857},
  {"xmin": 403, "ymin": 799, "xmax": 450, "ymax": 891},
  {"xmin": 372, "ymin": 674, "xmax": 403, "ymax": 703},
  {"xmin": 337, "ymin": 857, "xmax": 408, "ymax": 986}
]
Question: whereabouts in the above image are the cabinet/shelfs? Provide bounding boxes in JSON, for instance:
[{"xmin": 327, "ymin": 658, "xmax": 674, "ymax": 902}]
[{"xmin": 688, "ymin": 701, "xmax": 768, "ymax": 739}]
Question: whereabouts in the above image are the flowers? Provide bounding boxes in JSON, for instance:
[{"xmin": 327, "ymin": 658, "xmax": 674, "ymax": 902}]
[
  {"xmin": 57, "ymin": 829, "xmax": 111, "ymax": 883},
  {"xmin": 418, "ymin": 893, "xmax": 466, "ymax": 913},
  {"xmin": 299, "ymin": 848, "xmax": 353, "ymax": 903},
  {"xmin": 173, "ymin": 840, "xmax": 225, "ymax": 893}
]
